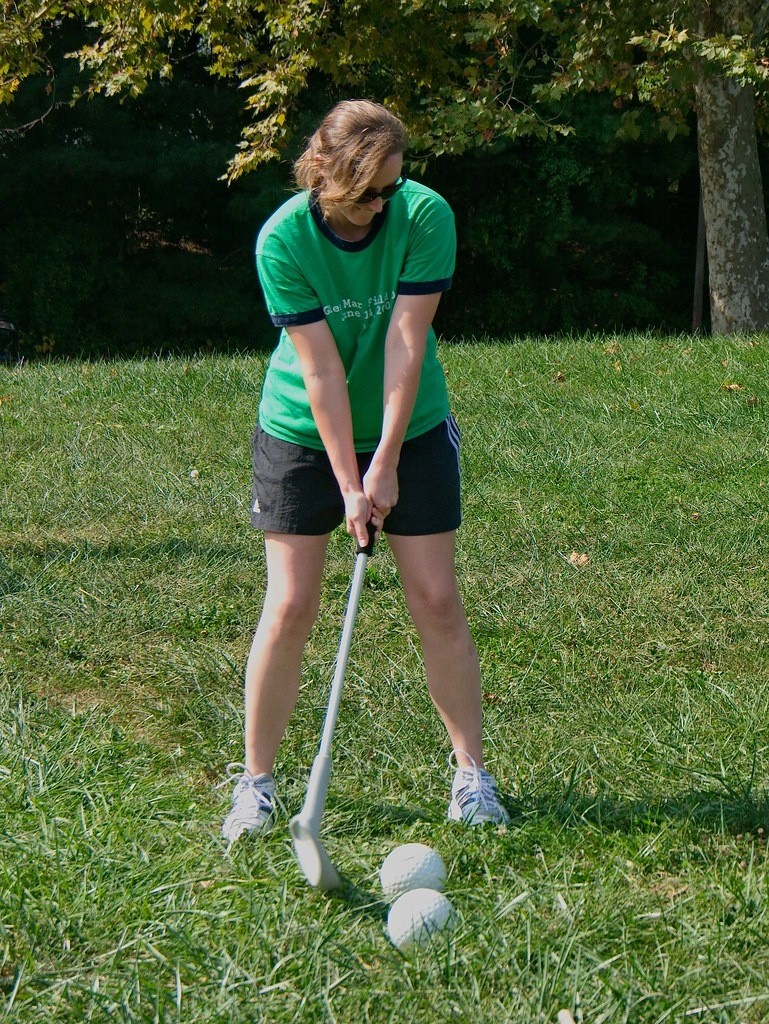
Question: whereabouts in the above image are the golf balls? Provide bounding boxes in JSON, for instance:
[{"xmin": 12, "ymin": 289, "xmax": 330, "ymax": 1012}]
[
  {"xmin": 380, "ymin": 842, "xmax": 448, "ymax": 896},
  {"xmin": 386, "ymin": 887, "xmax": 458, "ymax": 958}
]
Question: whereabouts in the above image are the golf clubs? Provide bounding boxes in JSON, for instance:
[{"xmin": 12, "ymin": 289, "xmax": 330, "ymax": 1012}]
[{"xmin": 290, "ymin": 523, "xmax": 377, "ymax": 889}]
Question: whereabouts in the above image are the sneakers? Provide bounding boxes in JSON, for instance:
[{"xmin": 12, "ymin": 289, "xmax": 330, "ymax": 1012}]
[
  {"xmin": 215, "ymin": 763, "xmax": 278, "ymax": 842},
  {"xmin": 448, "ymin": 749, "xmax": 511, "ymax": 827}
]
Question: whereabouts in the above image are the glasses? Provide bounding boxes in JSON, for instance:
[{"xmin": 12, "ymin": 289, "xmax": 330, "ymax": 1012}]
[{"xmin": 355, "ymin": 165, "xmax": 406, "ymax": 204}]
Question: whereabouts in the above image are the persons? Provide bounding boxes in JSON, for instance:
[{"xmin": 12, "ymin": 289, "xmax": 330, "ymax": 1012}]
[{"xmin": 212, "ymin": 98, "xmax": 513, "ymax": 845}]
